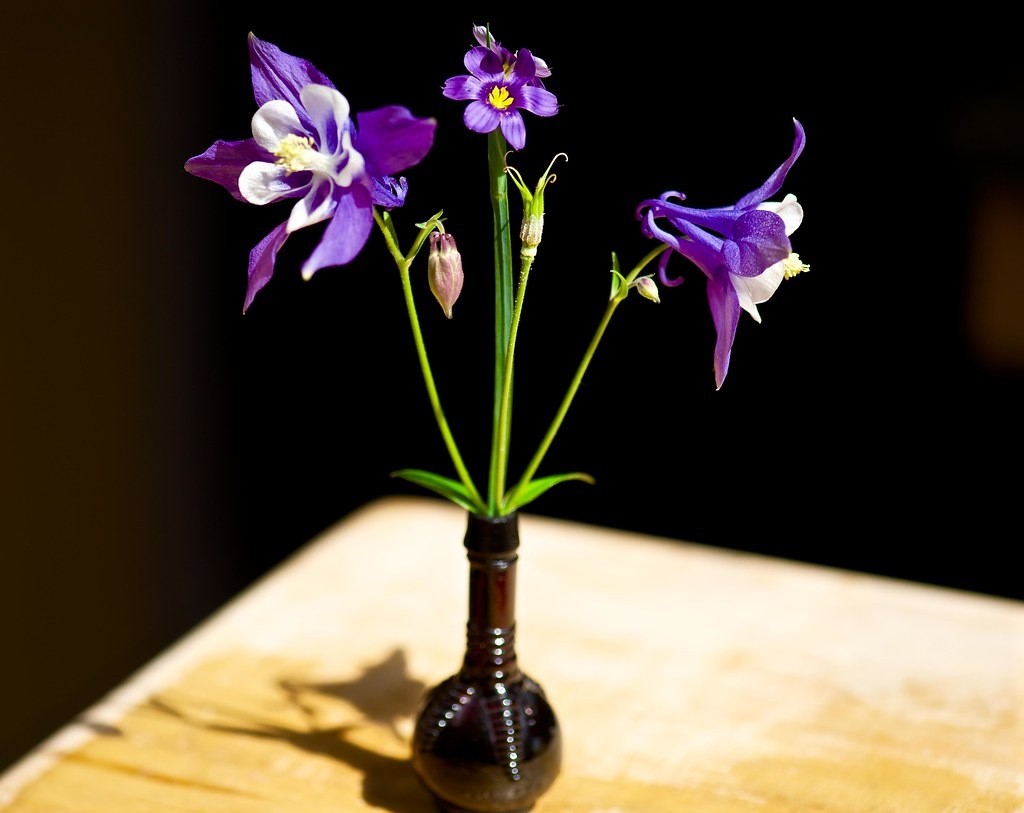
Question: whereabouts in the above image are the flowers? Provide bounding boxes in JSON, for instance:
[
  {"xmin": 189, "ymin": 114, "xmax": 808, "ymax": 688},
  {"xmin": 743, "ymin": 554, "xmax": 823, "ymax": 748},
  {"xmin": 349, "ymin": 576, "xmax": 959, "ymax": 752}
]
[{"xmin": 185, "ymin": 21, "xmax": 811, "ymax": 518}]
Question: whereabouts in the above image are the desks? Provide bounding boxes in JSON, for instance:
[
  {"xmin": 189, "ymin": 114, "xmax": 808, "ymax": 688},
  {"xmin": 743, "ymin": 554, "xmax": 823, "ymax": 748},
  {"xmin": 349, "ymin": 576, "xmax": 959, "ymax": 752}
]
[{"xmin": 0, "ymin": 497, "xmax": 1024, "ymax": 813}]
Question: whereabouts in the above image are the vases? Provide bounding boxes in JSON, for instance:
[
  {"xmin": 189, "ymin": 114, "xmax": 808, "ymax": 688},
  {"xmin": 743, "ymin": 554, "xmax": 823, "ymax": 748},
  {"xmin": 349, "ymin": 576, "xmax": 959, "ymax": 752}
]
[{"xmin": 410, "ymin": 510, "xmax": 562, "ymax": 813}]
